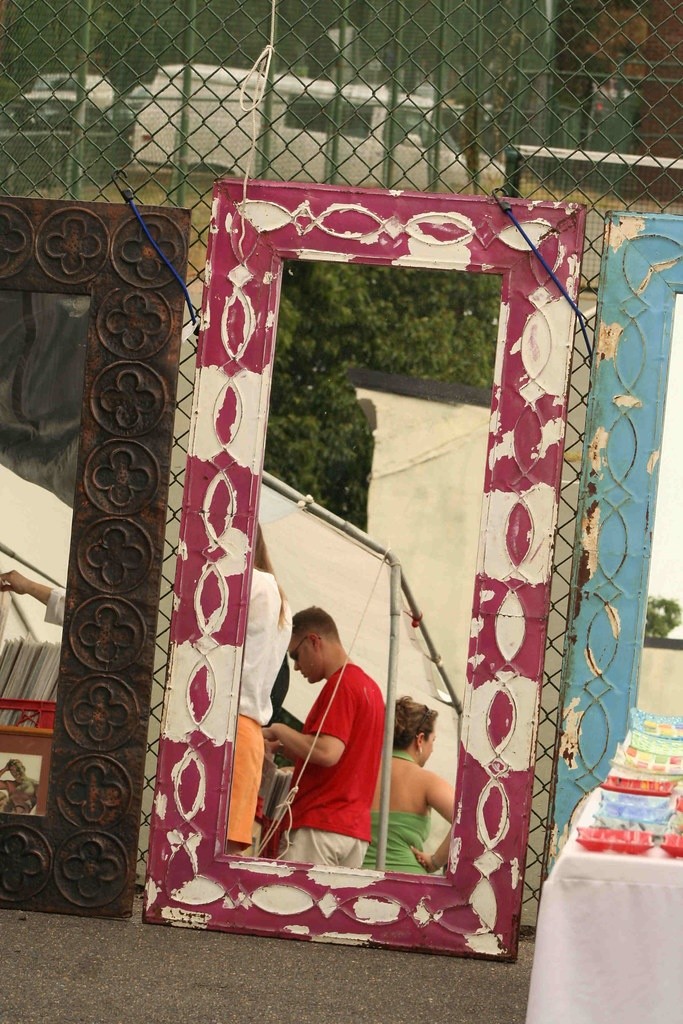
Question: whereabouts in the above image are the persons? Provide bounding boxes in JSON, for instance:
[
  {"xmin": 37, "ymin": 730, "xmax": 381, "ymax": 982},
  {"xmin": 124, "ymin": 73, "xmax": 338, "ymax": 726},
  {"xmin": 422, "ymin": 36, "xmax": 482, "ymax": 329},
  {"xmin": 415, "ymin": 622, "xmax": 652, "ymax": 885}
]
[
  {"xmin": 225, "ymin": 520, "xmax": 293, "ymax": 857},
  {"xmin": 360, "ymin": 696, "xmax": 456, "ymax": 875},
  {"xmin": 262, "ymin": 605, "xmax": 387, "ymax": 869},
  {"xmin": 1, "ymin": 571, "xmax": 66, "ymax": 626}
]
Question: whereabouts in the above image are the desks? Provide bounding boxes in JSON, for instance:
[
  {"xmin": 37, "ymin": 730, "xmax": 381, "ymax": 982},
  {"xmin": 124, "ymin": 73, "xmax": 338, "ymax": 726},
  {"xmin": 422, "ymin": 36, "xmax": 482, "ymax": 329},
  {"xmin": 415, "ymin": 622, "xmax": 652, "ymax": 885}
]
[{"xmin": 526, "ymin": 788, "xmax": 683, "ymax": 1024}]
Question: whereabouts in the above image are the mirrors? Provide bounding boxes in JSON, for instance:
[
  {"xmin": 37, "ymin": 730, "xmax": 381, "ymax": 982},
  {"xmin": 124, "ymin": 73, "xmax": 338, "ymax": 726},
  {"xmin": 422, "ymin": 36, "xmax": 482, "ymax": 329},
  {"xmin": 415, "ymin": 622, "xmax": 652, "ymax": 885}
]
[
  {"xmin": 0, "ymin": 196, "xmax": 193, "ymax": 921},
  {"xmin": 141, "ymin": 178, "xmax": 588, "ymax": 962},
  {"xmin": 548, "ymin": 210, "xmax": 683, "ymax": 873}
]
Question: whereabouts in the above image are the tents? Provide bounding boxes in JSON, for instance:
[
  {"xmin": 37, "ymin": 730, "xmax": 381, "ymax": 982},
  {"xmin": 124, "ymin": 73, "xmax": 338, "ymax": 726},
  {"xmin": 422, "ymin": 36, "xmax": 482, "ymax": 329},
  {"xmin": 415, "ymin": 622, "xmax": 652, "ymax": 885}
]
[{"xmin": 259, "ymin": 466, "xmax": 467, "ymax": 872}]
